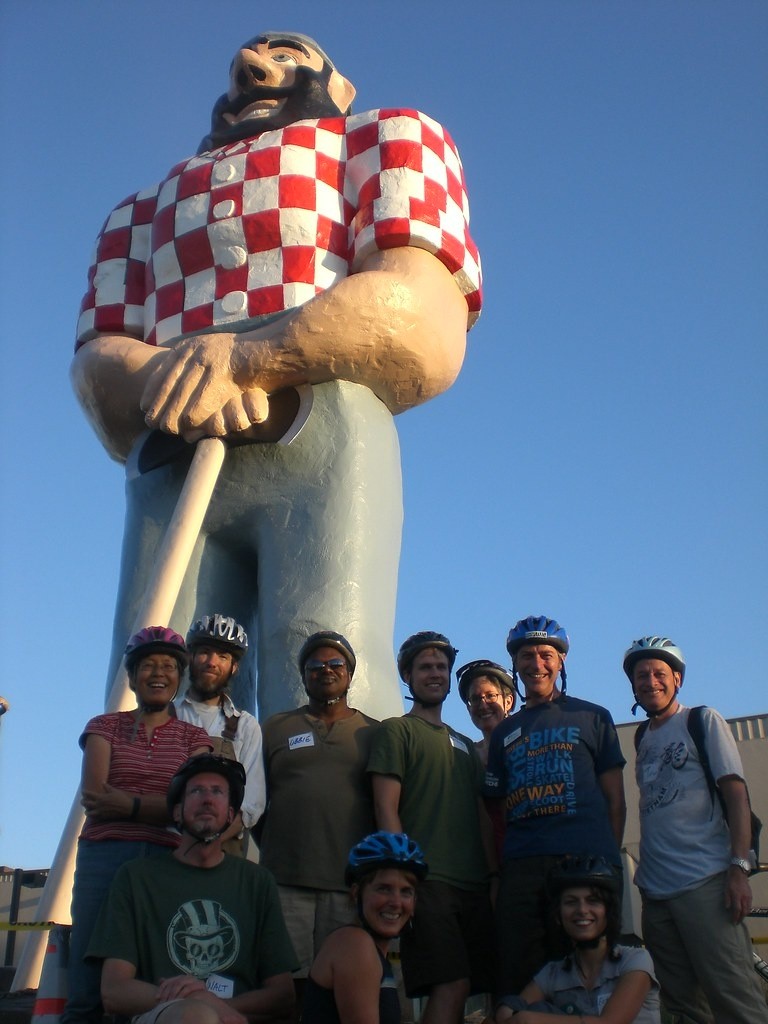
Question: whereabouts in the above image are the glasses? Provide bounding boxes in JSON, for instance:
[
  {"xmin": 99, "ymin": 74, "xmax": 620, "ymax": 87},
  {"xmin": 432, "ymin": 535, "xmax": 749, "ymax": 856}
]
[
  {"xmin": 304, "ymin": 658, "xmax": 346, "ymax": 672},
  {"xmin": 465, "ymin": 691, "xmax": 508, "ymax": 705},
  {"xmin": 140, "ymin": 662, "xmax": 179, "ymax": 672}
]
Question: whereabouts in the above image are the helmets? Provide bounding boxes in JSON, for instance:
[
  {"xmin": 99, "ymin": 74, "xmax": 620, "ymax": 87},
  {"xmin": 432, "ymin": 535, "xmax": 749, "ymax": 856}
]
[
  {"xmin": 124, "ymin": 626, "xmax": 187, "ymax": 669},
  {"xmin": 297, "ymin": 631, "xmax": 356, "ymax": 675},
  {"xmin": 165, "ymin": 753, "xmax": 246, "ymax": 829},
  {"xmin": 506, "ymin": 615, "xmax": 570, "ymax": 652},
  {"xmin": 551, "ymin": 847, "xmax": 620, "ymax": 886},
  {"xmin": 186, "ymin": 614, "xmax": 249, "ymax": 659},
  {"xmin": 455, "ymin": 659, "xmax": 518, "ymax": 712},
  {"xmin": 345, "ymin": 832, "xmax": 430, "ymax": 884},
  {"xmin": 396, "ymin": 631, "xmax": 457, "ymax": 683},
  {"xmin": 622, "ymin": 635, "xmax": 687, "ymax": 678}
]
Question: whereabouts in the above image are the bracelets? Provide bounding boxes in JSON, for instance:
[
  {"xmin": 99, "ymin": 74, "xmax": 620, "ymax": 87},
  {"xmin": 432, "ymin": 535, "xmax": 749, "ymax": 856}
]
[{"xmin": 130, "ymin": 797, "xmax": 141, "ymax": 819}]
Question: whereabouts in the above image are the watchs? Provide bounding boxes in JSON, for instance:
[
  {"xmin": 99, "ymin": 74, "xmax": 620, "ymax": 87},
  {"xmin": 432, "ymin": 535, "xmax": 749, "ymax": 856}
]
[{"xmin": 730, "ymin": 856, "xmax": 751, "ymax": 875}]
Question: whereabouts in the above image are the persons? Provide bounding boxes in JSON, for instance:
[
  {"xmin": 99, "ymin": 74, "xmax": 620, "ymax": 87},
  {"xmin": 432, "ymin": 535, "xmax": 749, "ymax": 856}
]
[
  {"xmin": 623, "ymin": 636, "xmax": 768, "ymax": 1023},
  {"xmin": 70, "ymin": 29, "xmax": 481, "ymax": 726},
  {"xmin": 62, "ymin": 615, "xmax": 661, "ymax": 1024}
]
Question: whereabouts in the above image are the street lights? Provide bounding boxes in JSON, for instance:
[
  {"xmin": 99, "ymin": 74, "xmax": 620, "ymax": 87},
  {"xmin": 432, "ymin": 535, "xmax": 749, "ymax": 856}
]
[{"xmin": 5, "ymin": 869, "xmax": 50, "ymax": 966}]
